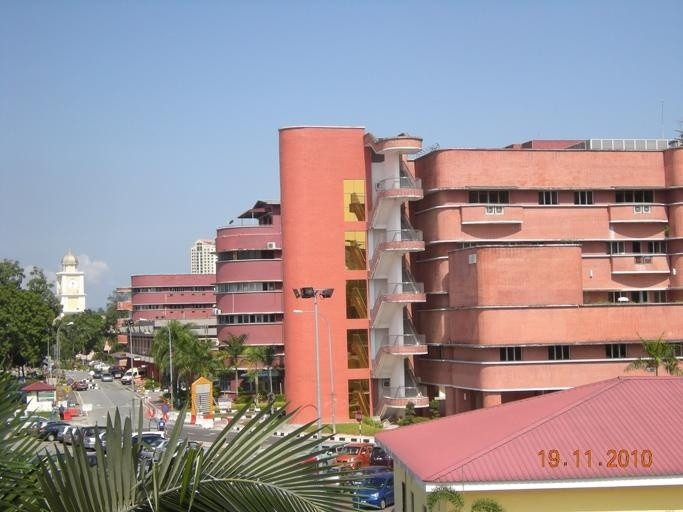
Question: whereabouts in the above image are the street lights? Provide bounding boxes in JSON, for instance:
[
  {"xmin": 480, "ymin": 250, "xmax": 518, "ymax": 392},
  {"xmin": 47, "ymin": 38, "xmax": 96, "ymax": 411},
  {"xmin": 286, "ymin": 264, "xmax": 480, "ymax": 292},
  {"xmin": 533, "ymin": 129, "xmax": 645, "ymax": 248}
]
[
  {"xmin": 46, "ymin": 312, "xmax": 74, "ymax": 379},
  {"xmin": 122, "ymin": 319, "xmax": 135, "ymax": 392},
  {"xmin": 139, "ymin": 317, "xmax": 173, "ymax": 412},
  {"xmin": 292, "ymin": 287, "xmax": 336, "ymax": 482}
]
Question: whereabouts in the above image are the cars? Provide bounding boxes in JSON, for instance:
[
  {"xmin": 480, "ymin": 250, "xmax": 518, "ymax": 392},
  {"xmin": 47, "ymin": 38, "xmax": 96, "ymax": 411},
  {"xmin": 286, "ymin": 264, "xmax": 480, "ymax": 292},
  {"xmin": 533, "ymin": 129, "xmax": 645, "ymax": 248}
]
[
  {"xmin": 71, "ymin": 360, "xmax": 146, "ymax": 391},
  {"xmin": 6, "ymin": 415, "xmax": 204, "ymax": 481},
  {"xmin": 298, "ymin": 441, "xmax": 394, "ymax": 510}
]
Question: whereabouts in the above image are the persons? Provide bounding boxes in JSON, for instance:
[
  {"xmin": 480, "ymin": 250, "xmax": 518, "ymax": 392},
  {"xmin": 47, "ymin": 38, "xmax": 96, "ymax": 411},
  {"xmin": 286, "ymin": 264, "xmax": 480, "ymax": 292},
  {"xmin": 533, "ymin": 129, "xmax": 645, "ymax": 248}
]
[
  {"xmin": 156, "ymin": 418, "xmax": 167, "ymax": 431},
  {"xmin": 162, "ymin": 401, "xmax": 169, "ymax": 421},
  {"xmin": 59, "ymin": 404, "xmax": 64, "ymax": 420}
]
